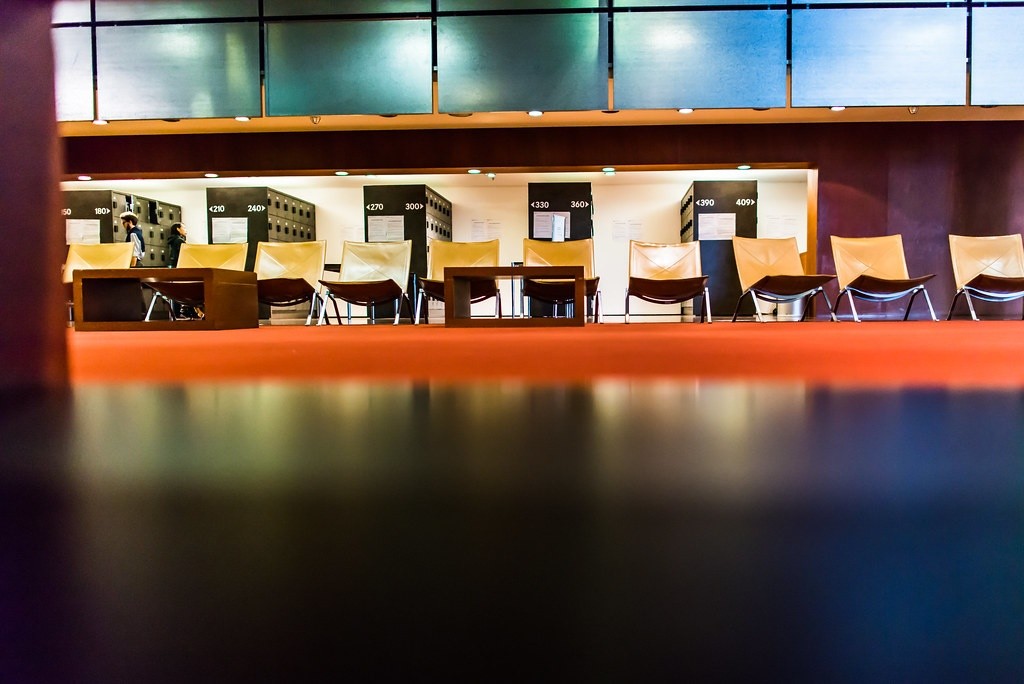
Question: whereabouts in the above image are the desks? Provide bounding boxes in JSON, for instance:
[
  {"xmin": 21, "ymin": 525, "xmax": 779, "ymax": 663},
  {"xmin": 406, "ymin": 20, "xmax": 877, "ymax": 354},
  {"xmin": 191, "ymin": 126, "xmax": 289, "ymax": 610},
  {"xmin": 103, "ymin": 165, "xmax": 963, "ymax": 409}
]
[
  {"xmin": 70, "ymin": 268, "xmax": 259, "ymax": 331},
  {"xmin": 443, "ymin": 265, "xmax": 585, "ymax": 328}
]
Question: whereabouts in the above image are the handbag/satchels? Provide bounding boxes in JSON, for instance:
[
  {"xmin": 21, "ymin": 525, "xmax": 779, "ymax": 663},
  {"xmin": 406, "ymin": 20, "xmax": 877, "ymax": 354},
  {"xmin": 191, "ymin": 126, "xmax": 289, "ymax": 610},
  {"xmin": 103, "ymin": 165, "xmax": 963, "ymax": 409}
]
[{"xmin": 169, "ymin": 297, "xmax": 204, "ymax": 320}]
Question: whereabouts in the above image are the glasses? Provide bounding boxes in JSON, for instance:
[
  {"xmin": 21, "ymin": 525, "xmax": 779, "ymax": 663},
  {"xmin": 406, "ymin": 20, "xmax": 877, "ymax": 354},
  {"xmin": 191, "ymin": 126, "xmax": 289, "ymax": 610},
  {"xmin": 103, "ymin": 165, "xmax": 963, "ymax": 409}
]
[{"xmin": 123, "ymin": 221, "xmax": 127, "ymax": 225}]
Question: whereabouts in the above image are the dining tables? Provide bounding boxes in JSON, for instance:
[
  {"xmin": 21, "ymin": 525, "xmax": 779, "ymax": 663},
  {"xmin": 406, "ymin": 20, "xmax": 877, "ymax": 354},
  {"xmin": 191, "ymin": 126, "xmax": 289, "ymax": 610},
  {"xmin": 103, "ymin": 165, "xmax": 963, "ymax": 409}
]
[{"xmin": 318, "ymin": 237, "xmax": 414, "ymax": 327}]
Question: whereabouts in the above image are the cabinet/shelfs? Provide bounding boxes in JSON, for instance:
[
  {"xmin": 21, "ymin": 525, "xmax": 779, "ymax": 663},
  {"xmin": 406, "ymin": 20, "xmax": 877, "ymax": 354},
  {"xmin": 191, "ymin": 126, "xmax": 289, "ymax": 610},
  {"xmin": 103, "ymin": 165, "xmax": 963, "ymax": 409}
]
[
  {"xmin": 678, "ymin": 179, "xmax": 761, "ymax": 315},
  {"xmin": 62, "ymin": 190, "xmax": 182, "ymax": 266},
  {"xmin": 527, "ymin": 182, "xmax": 598, "ymax": 319},
  {"xmin": 358, "ymin": 185, "xmax": 453, "ymax": 325},
  {"xmin": 205, "ymin": 186, "xmax": 316, "ymax": 320}
]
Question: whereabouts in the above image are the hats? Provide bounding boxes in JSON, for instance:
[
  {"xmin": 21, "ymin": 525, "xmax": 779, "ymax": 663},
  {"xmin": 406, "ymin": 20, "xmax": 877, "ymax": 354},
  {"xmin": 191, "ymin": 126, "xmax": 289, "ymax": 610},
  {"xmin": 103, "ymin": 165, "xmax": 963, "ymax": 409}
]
[{"xmin": 120, "ymin": 211, "xmax": 138, "ymax": 219}]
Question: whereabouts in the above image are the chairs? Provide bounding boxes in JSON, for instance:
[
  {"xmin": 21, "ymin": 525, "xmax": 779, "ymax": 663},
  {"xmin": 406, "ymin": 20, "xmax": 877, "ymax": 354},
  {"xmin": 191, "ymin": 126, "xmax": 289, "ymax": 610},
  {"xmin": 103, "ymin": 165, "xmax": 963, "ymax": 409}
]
[
  {"xmin": 828, "ymin": 233, "xmax": 940, "ymax": 321},
  {"xmin": 522, "ymin": 238, "xmax": 599, "ymax": 321},
  {"xmin": 946, "ymin": 232, "xmax": 1024, "ymax": 323},
  {"xmin": 624, "ymin": 240, "xmax": 713, "ymax": 323},
  {"xmin": 62, "ymin": 241, "xmax": 134, "ymax": 325},
  {"xmin": 251, "ymin": 239, "xmax": 330, "ymax": 325},
  {"xmin": 141, "ymin": 239, "xmax": 247, "ymax": 324},
  {"xmin": 414, "ymin": 241, "xmax": 504, "ymax": 325},
  {"xmin": 728, "ymin": 237, "xmax": 835, "ymax": 322}
]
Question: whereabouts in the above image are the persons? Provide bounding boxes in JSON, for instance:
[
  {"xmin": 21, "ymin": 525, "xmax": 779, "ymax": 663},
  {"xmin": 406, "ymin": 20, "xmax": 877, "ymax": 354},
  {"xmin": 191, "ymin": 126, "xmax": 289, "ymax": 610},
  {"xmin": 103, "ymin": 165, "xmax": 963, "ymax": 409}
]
[
  {"xmin": 121, "ymin": 211, "xmax": 146, "ymax": 267},
  {"xmin": 167, "ymin": 222, "xmax": 188, "ymax": 318}
]
